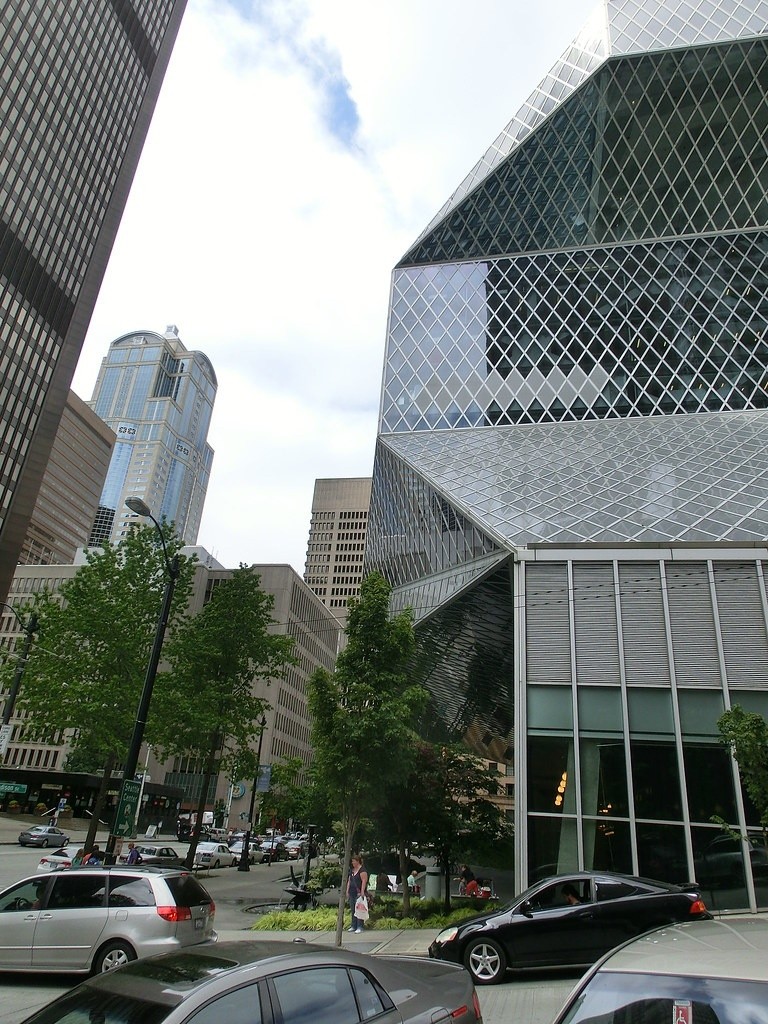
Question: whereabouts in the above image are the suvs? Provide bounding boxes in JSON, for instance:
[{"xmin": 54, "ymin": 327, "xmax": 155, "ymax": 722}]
[{"xmin": 0, "ymin": 862, "xmax": 217, "ymax": 979}]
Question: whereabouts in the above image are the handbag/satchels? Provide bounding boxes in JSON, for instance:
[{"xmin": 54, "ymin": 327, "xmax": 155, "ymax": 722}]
[
  {"xmin": 358, "ymin": 893, "xmax": 369, "ymax": 901},
  {"xmin": 354, "ymin": 896, "xmax": 369, "ymax": 921}
]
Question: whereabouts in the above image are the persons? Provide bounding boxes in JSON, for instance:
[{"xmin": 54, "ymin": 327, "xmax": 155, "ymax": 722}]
[
  {"xmin": 48, "ymin": 815, "xmax": 59, "ymax": 827},
  {"xmin": 376, "ymin": 866, "xmax": 393, "ymax": 891},
  {"xmin": 560, "ymin": 884, "xmax": 585, "ymax": 905},
  {"xmin": 452, "ymin": 865, "xmax": 483, "ymax": 895},
  {"xmin": 345, "ymin": 855, "xmax": 368, "ymax": 934},
  {"xmin": 406, "ymin": 870, "xmax": 417, "ymax": 893},
  {"xmin": 124, "ymin": 841, "xmax": 140, "ymax": 865},
  {"xmin": 70, "ymin": 845, "xmax": 104, "ymax": 866}
]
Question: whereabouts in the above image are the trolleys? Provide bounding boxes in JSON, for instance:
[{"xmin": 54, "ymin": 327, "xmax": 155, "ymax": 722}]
[{"xmin": 283, "ymin": 887, "xmax": 322, "ymax": 911}]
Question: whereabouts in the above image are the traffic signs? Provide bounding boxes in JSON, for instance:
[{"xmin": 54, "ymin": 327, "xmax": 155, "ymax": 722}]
[{"xmin": 113, "ymin": 779, "xmax": 143, "ymax": 838}]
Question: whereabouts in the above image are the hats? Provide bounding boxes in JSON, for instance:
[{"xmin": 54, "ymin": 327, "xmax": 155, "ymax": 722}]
[{"xmin": 412, "ymin": 871, "xmax": 417, "ymax": 875}]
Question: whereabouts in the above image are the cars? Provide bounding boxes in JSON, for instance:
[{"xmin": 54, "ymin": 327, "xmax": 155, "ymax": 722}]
[
  {"xmin": 177, "ymin": 825, "xmax": 337, "ymax": 866},
  {"xmin": 692, "ymin": 832, "xmax": 768, "ymax": 887},
  {"xmin": 18, "ymin": 824, "xmax": 70, "ymax": 849},
  {"xmin": 427, "ymin": 868, "xmax": 717, "ymax": 988},
  {"xmin": 546, "ymin": 911, "xmax": 768, "ymax": 1024},
  {"xmin": 35, "ymin": 846, "xmax": 85, "ymax": 875},
  {"xmin": 119, "ymin": 844, "xmax": 187, "ymax": 866},
  {"xmin": 193, "ymin": 841, "xmax": 237, "ymax": 869},
  {"xmin": 17, "ymin": 937, "xmax": 484, "ymax": 1024}
]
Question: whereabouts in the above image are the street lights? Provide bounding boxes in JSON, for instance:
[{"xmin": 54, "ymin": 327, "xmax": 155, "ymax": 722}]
[
  {"xmin": 234, "ymin": 713, "xmax": 267, "ymax": 872},
  {"xmin": 101, "ymin": 494, "xmax": 178, "ymax": 863}
]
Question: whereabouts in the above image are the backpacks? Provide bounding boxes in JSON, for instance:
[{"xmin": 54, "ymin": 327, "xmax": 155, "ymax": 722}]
[
  {"xmin": 131, "ymin": 850, "xmax": 143, "ymax": 865},
  {"xmin": 85, "ymin": 859, "xmax": 97, "ymax": 866}
]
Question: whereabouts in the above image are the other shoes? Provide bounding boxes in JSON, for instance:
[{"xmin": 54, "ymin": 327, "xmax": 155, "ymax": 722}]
[
  {"xmin": 354, "ymin": 929, "xmax": 364, "ymax": 933},
  {"xmin": 348, "ymin": 927, "xmax": 357, "ymax": 932}
]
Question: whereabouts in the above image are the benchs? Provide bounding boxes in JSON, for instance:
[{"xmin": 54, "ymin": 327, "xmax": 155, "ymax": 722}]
[
  {"xmin": 370, "ymin": 890, "xmax": 419, "ymax": 899},
  {"xmin": 451, "ymin": 895, "xmax": 500, "ymax": 905}
]
